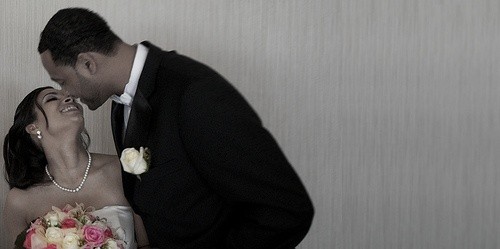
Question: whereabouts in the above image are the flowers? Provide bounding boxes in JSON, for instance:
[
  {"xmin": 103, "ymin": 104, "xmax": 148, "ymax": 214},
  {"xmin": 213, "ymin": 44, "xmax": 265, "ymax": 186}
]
[
  {"xmin": 14, "ymin": 202, "xmax": 127, "ymax": 249},
  {"xmin": 120, "ymin": 146, "xmax": 151, "ymax": 183}
]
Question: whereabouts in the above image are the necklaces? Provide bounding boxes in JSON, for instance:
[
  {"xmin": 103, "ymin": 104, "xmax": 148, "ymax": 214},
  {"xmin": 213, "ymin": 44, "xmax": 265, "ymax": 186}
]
[{"xmin": 45, "ymin": 149, "xmax": 92, "ymax": 193}]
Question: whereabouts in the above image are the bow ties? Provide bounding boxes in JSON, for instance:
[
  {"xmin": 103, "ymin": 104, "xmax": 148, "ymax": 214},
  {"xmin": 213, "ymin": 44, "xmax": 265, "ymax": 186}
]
[{"xmin": 110, "ymin": 92, "xmax": 133, "ymax": 106}]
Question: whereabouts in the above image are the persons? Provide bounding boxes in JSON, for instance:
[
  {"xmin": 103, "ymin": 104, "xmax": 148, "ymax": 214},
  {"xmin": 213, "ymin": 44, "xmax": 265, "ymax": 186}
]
[
  {"xmin": 1, "ymin": 86, "xmax": 150, "ymax": 249},
  {"xmin": 38, "ymin": 7, "xmax": 314, "ymax": 249}
]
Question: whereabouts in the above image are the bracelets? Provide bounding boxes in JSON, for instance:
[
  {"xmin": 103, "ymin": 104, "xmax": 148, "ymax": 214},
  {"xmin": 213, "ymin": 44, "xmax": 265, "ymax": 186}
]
[{"xmin": 137, "ymin": 244, "xmax": 150, "ymax": 249}]
